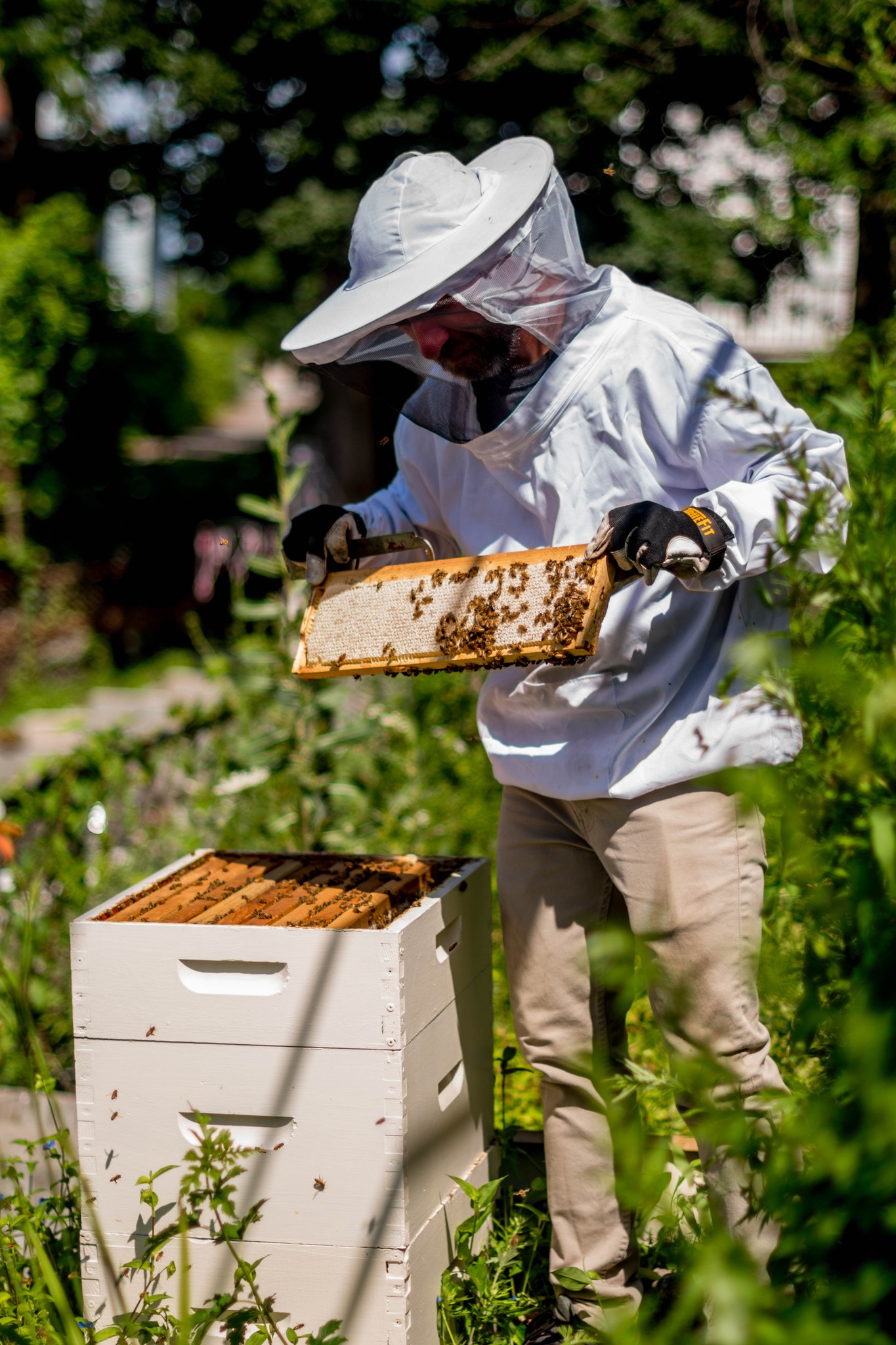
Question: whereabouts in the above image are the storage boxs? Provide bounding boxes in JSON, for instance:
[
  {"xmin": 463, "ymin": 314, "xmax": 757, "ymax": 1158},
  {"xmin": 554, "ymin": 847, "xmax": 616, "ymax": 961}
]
[{"xmin": 70, "ymin": 850, "xmax": 497, "ymax": 1345}]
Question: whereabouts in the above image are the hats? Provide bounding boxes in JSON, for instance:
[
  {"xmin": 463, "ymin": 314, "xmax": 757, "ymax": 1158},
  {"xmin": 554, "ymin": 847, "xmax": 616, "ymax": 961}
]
[{"xmin": 280, "ymin": 136, "xmax": 554, "ymax": 350}]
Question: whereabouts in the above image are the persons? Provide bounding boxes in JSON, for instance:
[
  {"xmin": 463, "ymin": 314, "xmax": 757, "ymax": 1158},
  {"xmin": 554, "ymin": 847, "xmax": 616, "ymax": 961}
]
[{"xmin": 281, "ymin": 135, "xmax": 851, "ymax": 1345}]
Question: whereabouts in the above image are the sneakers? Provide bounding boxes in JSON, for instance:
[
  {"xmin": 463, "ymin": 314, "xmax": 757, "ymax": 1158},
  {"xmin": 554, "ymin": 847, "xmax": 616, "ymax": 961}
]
[{"xmin": 521, "ymin": 1285, "xmax": 650, "ymax": 1345}]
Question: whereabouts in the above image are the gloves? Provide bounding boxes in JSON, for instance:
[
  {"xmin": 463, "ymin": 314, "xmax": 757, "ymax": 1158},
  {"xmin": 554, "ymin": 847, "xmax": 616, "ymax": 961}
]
[
  {"xmin": 280, "ymin": 503, "xmax": 370, "ymax": 590},
  {"xmin": 587, "ymin": 499, "xmax": 734, "ymax": 585}
]
